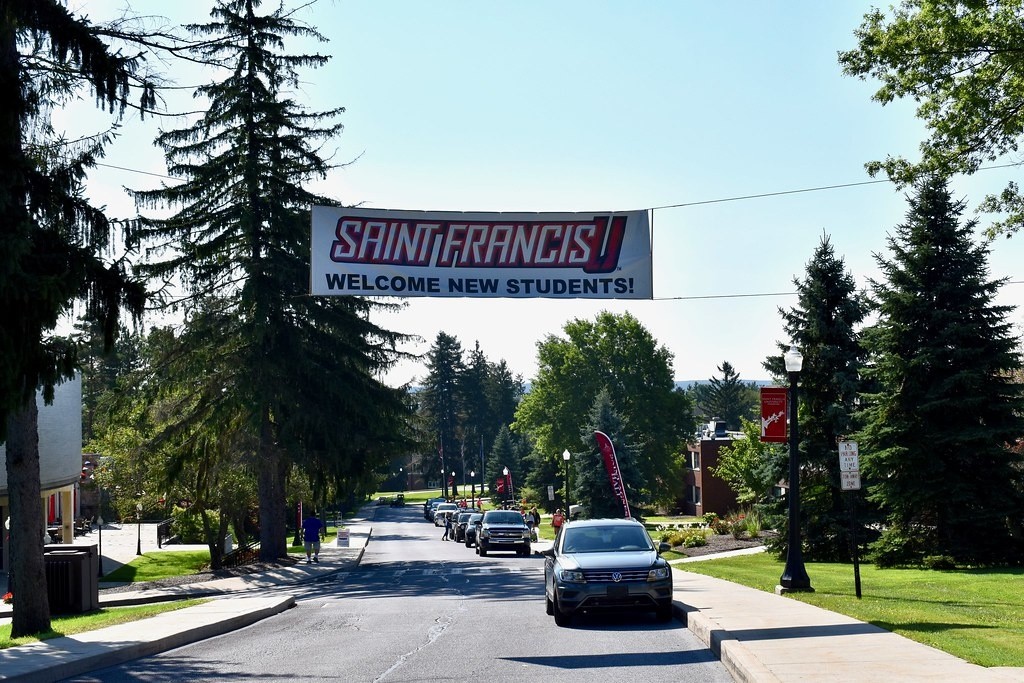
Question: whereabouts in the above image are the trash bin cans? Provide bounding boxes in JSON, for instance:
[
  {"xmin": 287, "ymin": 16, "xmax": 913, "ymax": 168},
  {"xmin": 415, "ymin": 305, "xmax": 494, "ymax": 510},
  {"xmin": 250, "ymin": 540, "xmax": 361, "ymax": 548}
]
[
  {"xmin": 43, "ymin": 549, "xmax": 91, "ymax": 614},
  {"xmin": 44, "ymin": 543, "xmax": 100, "ymax": 609}
]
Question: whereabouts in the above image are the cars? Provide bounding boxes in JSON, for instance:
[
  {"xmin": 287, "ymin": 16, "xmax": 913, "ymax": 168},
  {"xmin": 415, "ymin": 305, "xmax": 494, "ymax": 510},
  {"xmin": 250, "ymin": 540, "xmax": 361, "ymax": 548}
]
[{"xmin": 422, "ymin": 498, "xmax": 484, "ymax": 548}]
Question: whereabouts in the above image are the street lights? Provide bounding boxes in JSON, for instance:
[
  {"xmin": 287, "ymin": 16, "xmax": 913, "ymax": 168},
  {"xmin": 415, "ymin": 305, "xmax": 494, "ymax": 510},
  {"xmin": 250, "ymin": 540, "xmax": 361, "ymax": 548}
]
[
  {"xmin": 470, "ymin": 470, "xmax": 475, "ymax": 509},
  {"xmin": 778, "ymin": 341, "xmax": 816, "ymax": 594},
  {"xmin": 562, "ymin": 448, "xmax": 571, "ymax": 520},
  {"xmin": 451, "ymin": 471, "xmax": 456, "ymax": 501},
  {"xmin": 503, "ymin": 467, "xmax": 509, "ymax": 510},
  {"xmin": 440, "ymin": 469, "xmax": 444, "ymax": 498},
  {"xmin": 97, "ymin": 515, "xmax": 105, "ymax": 578},
  {"xmin": 136, "ymin": 502, "xmax": 143, "ymax": 555}
]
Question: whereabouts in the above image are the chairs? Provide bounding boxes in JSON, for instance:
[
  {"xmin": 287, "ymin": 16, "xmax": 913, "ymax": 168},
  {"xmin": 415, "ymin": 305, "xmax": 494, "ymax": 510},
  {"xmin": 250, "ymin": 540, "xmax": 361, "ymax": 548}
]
[{"xmin": 41, "ymin": 515, "xmax": 95, "ymax": 542}]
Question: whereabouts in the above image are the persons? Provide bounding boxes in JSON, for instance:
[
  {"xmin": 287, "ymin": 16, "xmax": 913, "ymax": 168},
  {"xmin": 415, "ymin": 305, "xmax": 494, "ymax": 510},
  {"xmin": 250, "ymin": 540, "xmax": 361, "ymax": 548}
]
[
  {"xmin": 552, "ymin": 509, "xmax": 563, "ymax": 538},
  {"xmin": 459, "ymin": 499, "xmax": 468, "ymax": 508},
  {"xmin": 442, "ymin": 512, "xmax": 452, "ymax": 542},
  {"xmin": 477, "ymin": 497, "xmax": 483, "ymax": 511},
  {"xmin": 301, "ymin": 512, "xmax": 324, "ymax": 565},
  {"xmin": 510, "ymin": 506, "xmax": 541, "ymax": 542}
]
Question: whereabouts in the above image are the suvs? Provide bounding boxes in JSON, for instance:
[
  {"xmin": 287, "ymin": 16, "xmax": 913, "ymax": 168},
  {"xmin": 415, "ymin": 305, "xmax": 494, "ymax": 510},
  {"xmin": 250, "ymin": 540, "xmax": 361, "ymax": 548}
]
[
  {"xmin": 542, "ymin": 517, "xmax": 673, "ymax": 627},
  {"xmin": 474, "ymin": 509, "xmax": 531, "ymax": 558}
]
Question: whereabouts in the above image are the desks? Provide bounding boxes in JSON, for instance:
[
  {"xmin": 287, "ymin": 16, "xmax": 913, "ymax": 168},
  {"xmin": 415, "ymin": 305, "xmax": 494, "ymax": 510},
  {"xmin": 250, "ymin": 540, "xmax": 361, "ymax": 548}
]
[{"xmin": 48, "ymin": 528, "xmax": 58, "ymax": 541}]
